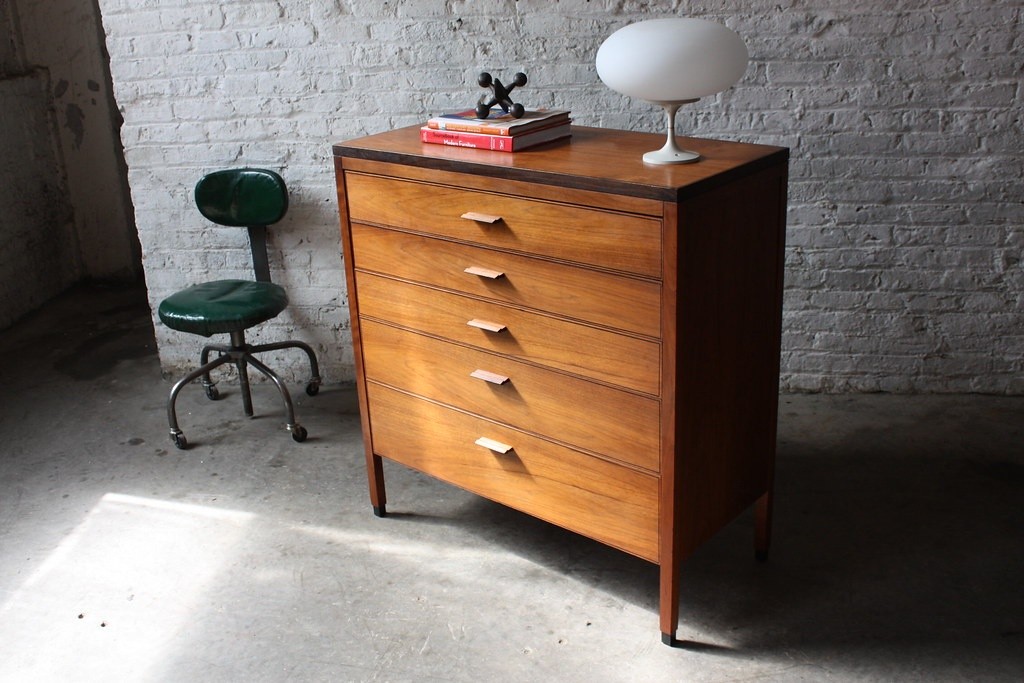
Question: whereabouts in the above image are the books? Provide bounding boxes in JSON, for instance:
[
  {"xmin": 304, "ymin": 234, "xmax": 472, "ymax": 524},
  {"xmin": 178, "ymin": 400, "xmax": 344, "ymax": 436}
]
[{"xmin": 420, "ymin": 108, "xmax": 573, "ymax": 153}]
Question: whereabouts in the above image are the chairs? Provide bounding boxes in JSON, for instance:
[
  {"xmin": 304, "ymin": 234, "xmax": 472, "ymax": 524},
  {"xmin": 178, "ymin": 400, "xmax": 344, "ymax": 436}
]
[{"xmin": 157, "ymin": 168, "xmax": 321, "ymax": 449}]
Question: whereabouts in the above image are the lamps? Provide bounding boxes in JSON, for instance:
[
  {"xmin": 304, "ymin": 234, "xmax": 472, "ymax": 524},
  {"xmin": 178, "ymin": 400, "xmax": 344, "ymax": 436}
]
[{"xmin": 596, "ymin": 17, "xmax": 749, "ymax": 165}]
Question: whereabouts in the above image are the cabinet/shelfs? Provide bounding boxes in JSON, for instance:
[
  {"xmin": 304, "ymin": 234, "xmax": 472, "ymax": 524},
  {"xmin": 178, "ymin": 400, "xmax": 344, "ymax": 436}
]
[{"xmin": 332, "ymin": 122, "xmax": 790, "ymax": 646}]
